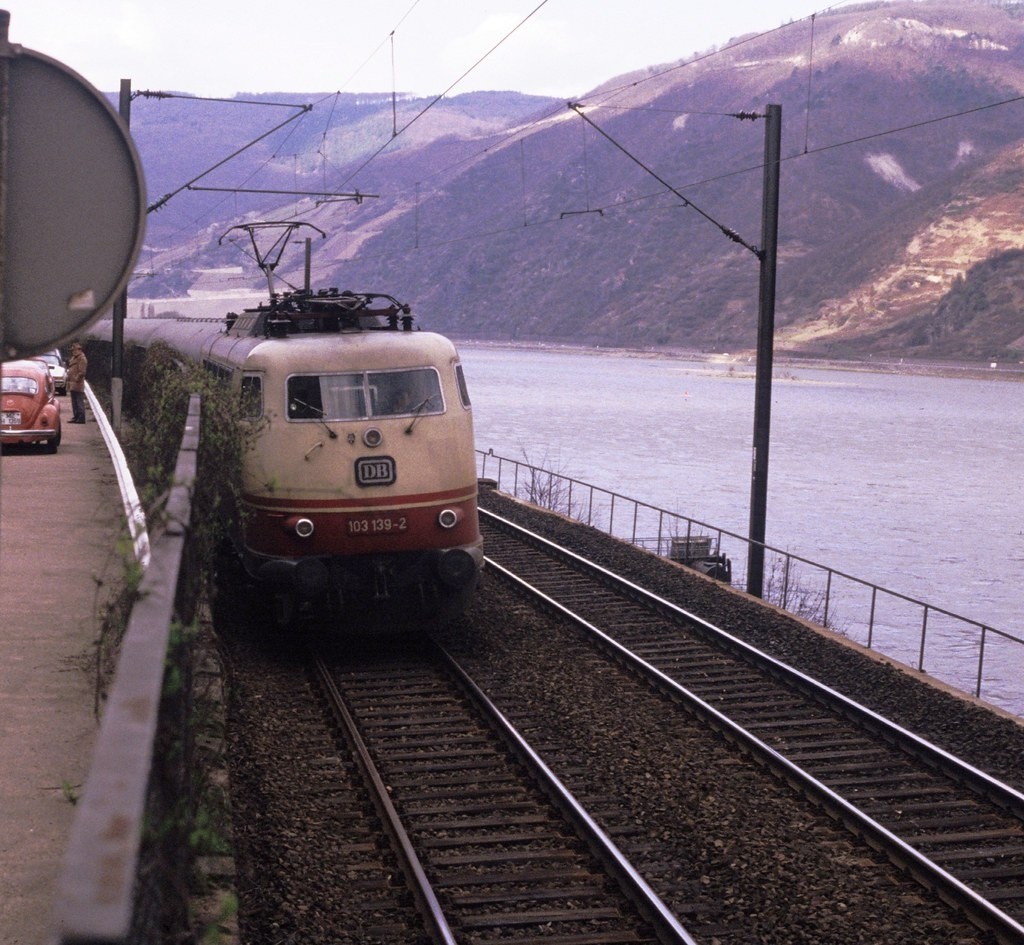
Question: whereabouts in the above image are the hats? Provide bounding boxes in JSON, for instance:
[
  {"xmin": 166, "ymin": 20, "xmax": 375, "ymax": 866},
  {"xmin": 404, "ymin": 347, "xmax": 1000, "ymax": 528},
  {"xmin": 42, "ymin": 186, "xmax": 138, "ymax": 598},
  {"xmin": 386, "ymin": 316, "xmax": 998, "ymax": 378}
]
[{"xmin": 68, "ymin": 343, "xmax": 81, "ymax": 350}]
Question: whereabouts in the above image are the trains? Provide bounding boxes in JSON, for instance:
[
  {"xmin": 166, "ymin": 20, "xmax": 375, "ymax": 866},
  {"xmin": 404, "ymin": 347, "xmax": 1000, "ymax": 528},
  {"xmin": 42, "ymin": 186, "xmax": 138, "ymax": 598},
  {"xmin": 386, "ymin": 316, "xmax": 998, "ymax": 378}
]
[{"xmin": 62, "ymin": 288, "xmax": 484, "ymax": 648}]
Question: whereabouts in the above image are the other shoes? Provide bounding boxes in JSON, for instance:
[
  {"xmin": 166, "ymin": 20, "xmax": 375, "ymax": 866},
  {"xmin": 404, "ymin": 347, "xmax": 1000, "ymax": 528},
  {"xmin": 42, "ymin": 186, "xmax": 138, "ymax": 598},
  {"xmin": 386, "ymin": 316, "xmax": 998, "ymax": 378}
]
[{"xmin": 67, "ymin": 418, "xmax": 86, "ymax": 424}]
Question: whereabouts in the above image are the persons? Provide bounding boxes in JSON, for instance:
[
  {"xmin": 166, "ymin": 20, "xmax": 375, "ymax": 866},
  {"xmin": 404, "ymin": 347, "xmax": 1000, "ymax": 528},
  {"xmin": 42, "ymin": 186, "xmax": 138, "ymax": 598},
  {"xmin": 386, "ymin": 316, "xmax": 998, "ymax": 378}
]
[
  {"xmin": 383, "ymin": 389, "xmax": 411, "ymax": 415},
  {"xmin": 66, "ymin": 342, "xmax": 87, "ymax": 424}
]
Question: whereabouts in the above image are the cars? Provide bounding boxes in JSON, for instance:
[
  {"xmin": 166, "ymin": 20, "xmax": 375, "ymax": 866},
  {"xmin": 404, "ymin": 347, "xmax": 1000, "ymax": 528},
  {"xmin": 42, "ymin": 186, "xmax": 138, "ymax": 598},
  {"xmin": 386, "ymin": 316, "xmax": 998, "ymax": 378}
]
[
  {"xmin": 38, "ymin": 350, "xmax": 67, "ymax": 394},
  {"xmin": 0, "ymin": 359, "xmax": 63, "ymax": 455}
]
